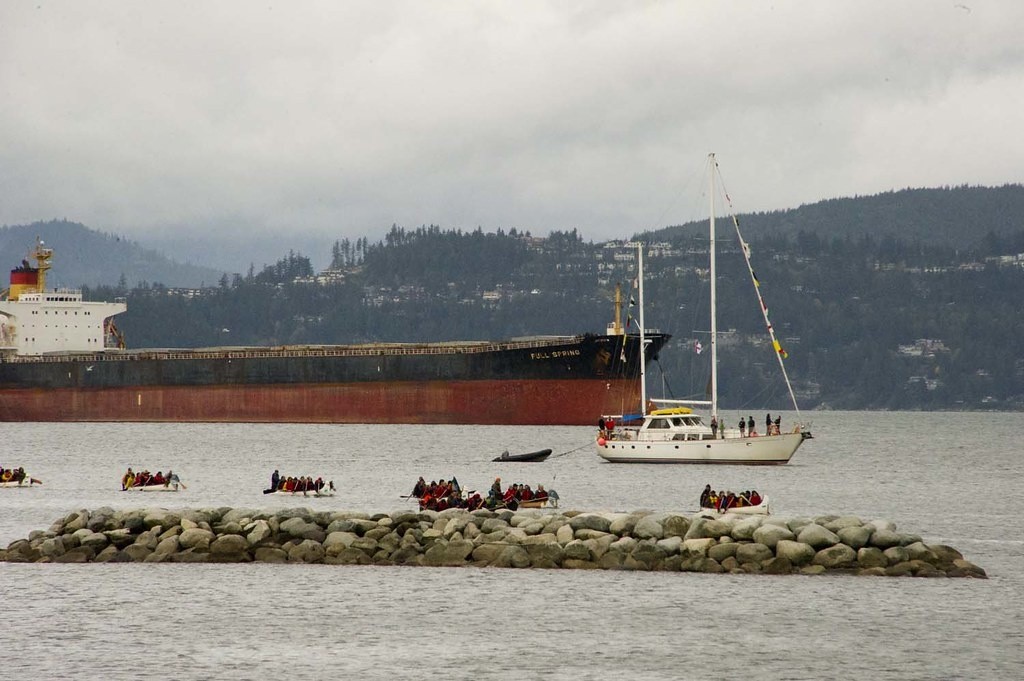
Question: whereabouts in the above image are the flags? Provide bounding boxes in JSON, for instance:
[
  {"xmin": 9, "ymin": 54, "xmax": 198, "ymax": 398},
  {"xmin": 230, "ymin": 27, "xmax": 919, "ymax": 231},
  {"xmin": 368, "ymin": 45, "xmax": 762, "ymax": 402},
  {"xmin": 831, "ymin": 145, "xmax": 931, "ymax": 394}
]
[
  {"xmin": 715, "ymin": 163, "xmax": 788, "ymax": 359},
  {"xmin": 620, "ymin": 279, "xmax": 639, "ymax": 362},
  {"xmin": 695, "ymin": 339, "xmax": 703, "ymax": 355}
]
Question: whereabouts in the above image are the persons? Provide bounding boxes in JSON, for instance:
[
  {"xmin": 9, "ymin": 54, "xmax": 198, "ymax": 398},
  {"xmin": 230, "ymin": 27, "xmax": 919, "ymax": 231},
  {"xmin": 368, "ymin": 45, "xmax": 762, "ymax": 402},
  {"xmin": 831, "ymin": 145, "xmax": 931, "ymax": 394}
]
[
  {"xmin": 0, "ymin": 466, "xmax": 26, "ymax": 485},
  {"xmin": 484, "ymin": 476, "xmax": 549, "ymax": 502},
  {"xmin": 700, "ymin": 484, "xmax": 763, "ymax": 514},
  {"xmin": 765, "ymin": 413, "xmax": 774, "ymax": 436},
  {"xmin": 748, "ymin": 416, "xmax": 755, "ymax": 437},
  {"xmin": 774, "ymin": 415, "xmax": 781, "ymax": 435},
  {"xmin": 412, "ymin": 475, "xmax": 452, "ymax": 499},
  {"xmin": 417, "ymin": 492, "xmax": 482, "ymax": 512},
  {"xmin": 738, "ymin": 417, "xmax": 746, "ymax": 438},
  {"xmin": 122, "ymin": 467, "xmax": 173, "ymax": 488},
  {"xmin": 711, "ymin": 418, "xmax": 718, "ymax": 435},
  {"xmin": 271, "ymin": 470, "xmax": 324, "ymax": 494},
  {"xmin": 598, "ymin": 414, "xmax": 615, "ymax": 440},
  {"xmin": 719, "ymin": 419, "xmax": 725, "ymax": 438}
]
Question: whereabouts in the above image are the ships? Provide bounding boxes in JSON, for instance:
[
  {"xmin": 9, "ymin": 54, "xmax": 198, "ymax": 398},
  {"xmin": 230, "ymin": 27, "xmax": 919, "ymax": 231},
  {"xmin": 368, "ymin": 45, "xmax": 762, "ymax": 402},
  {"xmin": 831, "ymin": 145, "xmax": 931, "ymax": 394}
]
[{"xmin": 0, "ymin": 236, "xmax": 673, "ymax": 426}]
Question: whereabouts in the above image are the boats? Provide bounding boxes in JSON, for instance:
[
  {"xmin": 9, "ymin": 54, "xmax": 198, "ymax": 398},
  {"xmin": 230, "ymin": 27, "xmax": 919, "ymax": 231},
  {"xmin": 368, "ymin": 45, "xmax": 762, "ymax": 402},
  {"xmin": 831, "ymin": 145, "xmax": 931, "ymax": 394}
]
[
  {"xmin": 492, "ymin": 448, "xmax": 552, "ymax": 462},
  {"xmin": 126, "ymin": 475, "xmax": 179, "ymax": 491},
  {"xmin": 401, "ymin": 478, "xmax": 560, "ymax": 511},
  {"xmin": 276, "ymin": 480, "xmax": 335, "ymax": 497},
  {"xmin": 0, "ymin": 474, "xmax": 32, "ymax": 488},
  {"xmin": 696, "ymin": 491, "xmax": 769, "ymax": 520}
]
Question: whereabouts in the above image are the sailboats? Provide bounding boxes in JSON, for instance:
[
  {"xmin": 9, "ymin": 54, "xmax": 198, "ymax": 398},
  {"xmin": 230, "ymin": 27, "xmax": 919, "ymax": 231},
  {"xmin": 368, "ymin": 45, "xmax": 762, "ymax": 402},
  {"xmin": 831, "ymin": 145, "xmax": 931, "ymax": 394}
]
[{"xmin": 595, "ymin": 153, "xmax": 815, "ymax": 464}]
